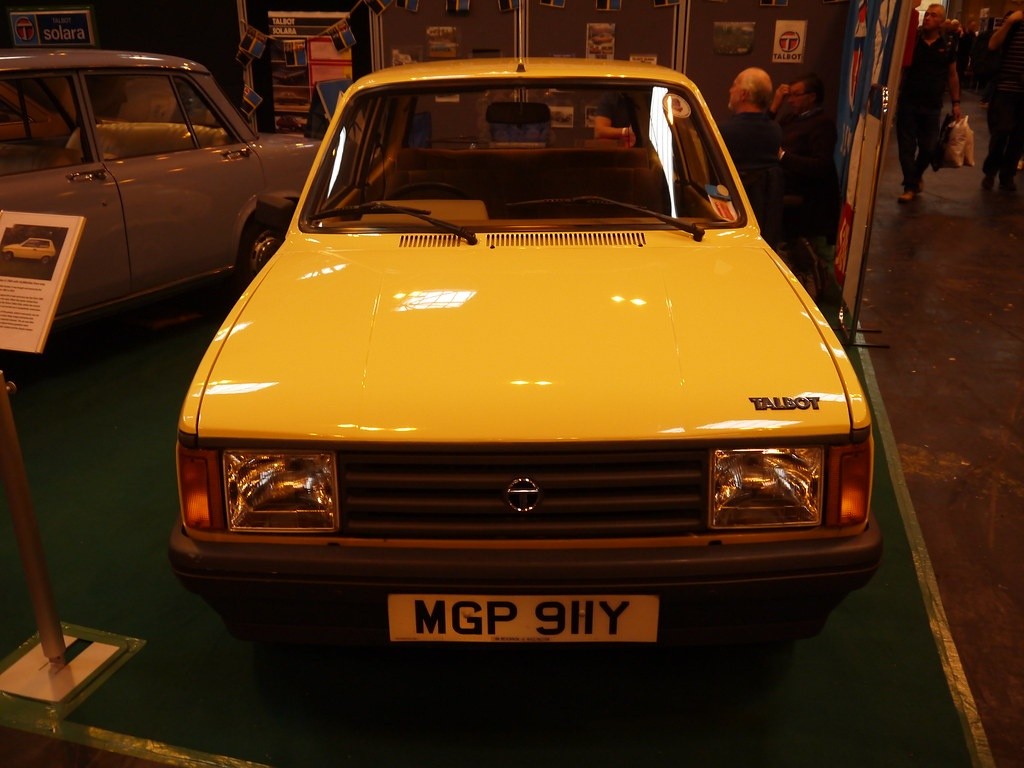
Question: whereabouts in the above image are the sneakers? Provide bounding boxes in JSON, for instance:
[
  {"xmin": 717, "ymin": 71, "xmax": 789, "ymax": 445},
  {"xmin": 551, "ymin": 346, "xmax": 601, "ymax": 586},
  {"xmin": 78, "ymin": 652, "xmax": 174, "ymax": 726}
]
[
  {"xmin": 898, "ymin": 190, "xmax": 916, "ymax": 203},
  {"xmin": 916, "ymin": 181, "xmax": 925, "ymax": 192}
]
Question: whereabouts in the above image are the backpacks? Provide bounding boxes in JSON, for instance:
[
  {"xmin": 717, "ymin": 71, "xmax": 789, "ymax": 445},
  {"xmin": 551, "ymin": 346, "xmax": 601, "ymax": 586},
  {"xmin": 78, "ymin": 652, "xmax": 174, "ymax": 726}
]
[{"xmin": 968, "ymin": 18, "xmax": 1020, "ymax": 104}]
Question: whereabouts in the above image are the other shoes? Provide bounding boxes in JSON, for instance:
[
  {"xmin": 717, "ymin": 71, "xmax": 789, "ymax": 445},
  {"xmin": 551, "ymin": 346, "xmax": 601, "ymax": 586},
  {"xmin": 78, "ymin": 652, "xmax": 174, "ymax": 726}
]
[
  {"xmin": 981, "ymin": 175, "xmax": 994, "ymax": 191},
  {"xmin": 998, "ymin": 179, "xmax": 1018, "ymax": 191}
]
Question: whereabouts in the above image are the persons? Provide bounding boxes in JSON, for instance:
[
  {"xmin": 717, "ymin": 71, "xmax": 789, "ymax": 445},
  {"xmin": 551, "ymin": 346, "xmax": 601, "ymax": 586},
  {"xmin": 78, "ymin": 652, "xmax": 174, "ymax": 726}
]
[
  {"xmin": 765, "ymin": 73, "xmax": 842, "ymax": 284},
  {"xmin": 981, "ymin": 1, "xmax": 1024, "ymax": 192},
  {"xmin": 714, "ymin": 67, "xmax": 786, "ymax": 189},
  {"xmin": 894, "ymin": 4, "xmax": 962, "ymax": 202},
  {"xmin": 941, "ymin": 16, "xmax": 998, "ymax": 86},
  {"xmin": 593, "ymin": 89, "xmax": 645, "ymax": 142}
]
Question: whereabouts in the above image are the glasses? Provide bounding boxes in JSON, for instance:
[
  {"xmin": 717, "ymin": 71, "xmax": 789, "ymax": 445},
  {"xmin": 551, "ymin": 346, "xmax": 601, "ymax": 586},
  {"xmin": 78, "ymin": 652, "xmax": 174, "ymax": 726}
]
[{"xmin": 788, "ymin": 91, "xmax": 807, "ymax": 97}]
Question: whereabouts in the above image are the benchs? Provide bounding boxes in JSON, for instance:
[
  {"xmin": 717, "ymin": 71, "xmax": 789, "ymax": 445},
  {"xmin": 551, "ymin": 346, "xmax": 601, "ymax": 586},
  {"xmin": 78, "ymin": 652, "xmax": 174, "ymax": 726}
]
[
  {"xmin": 395, "ymin": 147, "xmax": 643, "ymax": 205},
  {"xmin": 67, "ymin": 123, "xmax": 227, "ymax": 160}
]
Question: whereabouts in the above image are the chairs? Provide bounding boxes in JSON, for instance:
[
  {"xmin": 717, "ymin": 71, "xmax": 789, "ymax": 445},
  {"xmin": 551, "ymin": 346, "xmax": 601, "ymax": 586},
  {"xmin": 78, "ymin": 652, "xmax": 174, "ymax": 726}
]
[
  {"xmin": 381, "ymin": 165, "xmax": 490, "ymax": 206},
  {"xmin": 548, "ymin": 167, "xmax": 663, "ymax": 212},
  {"xmin": 0, "ymin": 145, "xmax": 81, "ymax": 176}
]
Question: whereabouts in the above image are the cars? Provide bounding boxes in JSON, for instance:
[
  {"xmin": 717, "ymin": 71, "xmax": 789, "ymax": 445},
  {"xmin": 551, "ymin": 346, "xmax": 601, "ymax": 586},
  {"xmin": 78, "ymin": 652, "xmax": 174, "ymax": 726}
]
[
  {"xmin": 169, "ymin": 56, "xmax": 884, "ymax": 646},
  {"xmin": 3, "ymin": 238, "xmax": 56, "ymax": 265},
  {"xmin": 0, "ymin": 47, "xmax": 324, "ymax": 334}
]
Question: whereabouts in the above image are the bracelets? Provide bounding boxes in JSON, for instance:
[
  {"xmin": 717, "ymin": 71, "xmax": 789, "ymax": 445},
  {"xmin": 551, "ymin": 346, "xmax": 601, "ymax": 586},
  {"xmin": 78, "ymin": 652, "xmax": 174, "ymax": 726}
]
[
  {"xmin": 621, "ymin": 127, "xmax": 628, "ymax": 138},
  {"xmin": 951, "ymin": 99, "xmax": 961, "ymax": 105}
]
